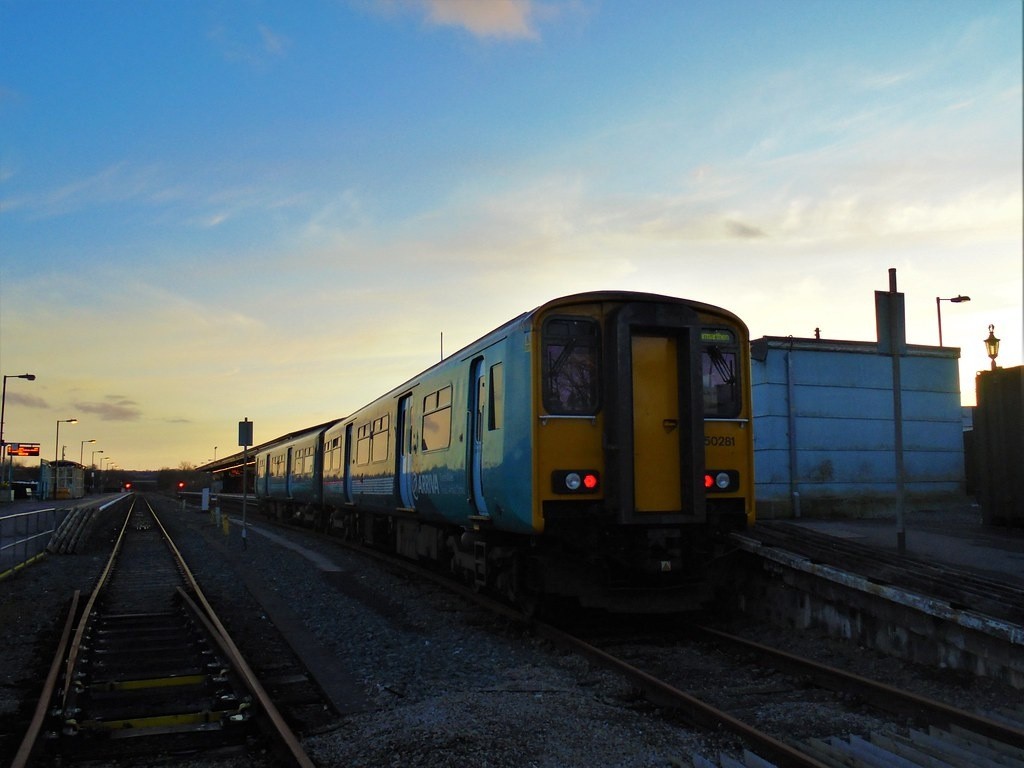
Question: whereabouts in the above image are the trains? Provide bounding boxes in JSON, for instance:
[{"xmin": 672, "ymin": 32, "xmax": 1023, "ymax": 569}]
[{"xmin": 249, "ymin": 288, "xmax": 759, "ymax": 623}]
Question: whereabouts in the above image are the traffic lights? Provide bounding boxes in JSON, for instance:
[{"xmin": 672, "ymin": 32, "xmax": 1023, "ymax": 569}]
[
  {"xmin": 126, "ymin": 483, "xmax": 132, "ymax": 489},
  {"xmin": 178, "ymin": 482, "xmax": 185, "ymax": 488}
]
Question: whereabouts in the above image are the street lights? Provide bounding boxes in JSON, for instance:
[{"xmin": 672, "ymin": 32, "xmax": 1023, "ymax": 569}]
[
  {"xmin": 100, "ymin": 458, "xmax": 109, "ymax": 471},
  {"xmin": 53, "ymin": 419, "xmax": 78, "ymax": 498},
  {"xmin": 112, "ymin": 465, "xmax": 118, "ymax": 471},
  {"xmin": 107, "ymin": 462, "xmax": 114, "ymax": 471},
  {"xmin": 983, "ymin": 324, "xmax": 1000, "ymax": 371},
  {"xmin": 0, "ymin": 373, "xmax": 37, "ymax": 460},
  {"xmin": 936, "ymin": 295, "xmax": 971, "ymax": 348},
  {"xmin": 92, "ymin": 450, "xmax": 104, "ymax": 471},
  {"xmin": 80, "ymin": 440, "xmax": 95, "ymax": 466}
]
